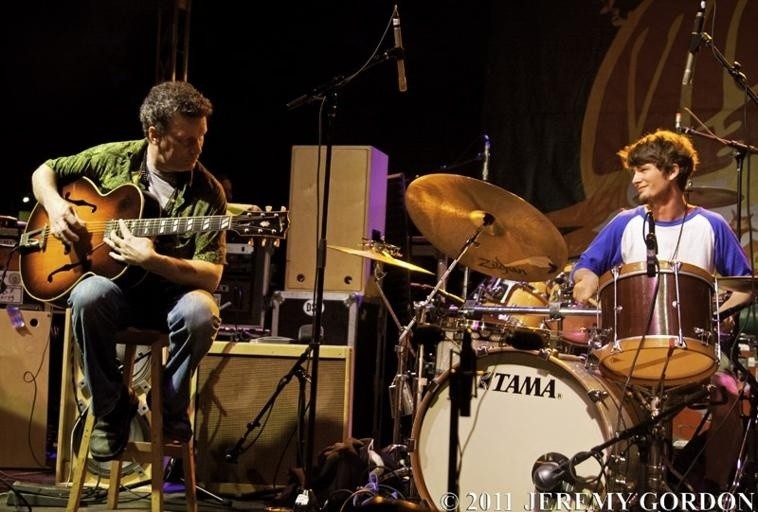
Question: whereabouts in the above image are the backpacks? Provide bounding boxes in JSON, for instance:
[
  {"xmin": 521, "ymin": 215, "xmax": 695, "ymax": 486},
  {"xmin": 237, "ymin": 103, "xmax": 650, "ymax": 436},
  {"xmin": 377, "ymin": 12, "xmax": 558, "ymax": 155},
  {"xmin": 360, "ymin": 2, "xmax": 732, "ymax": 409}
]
[
  {"xmin": 410, "ymin": 348, "xmax": 645, "ymax": 512},
  {"xmin": 544, "ymin": 263, "xmax": 608, "ymax": 346},
  {"xmin": 481, "ymin": 274, "xmax": 557, "ymax": 350},
  {"xmin": 596, "ymin": 262, "xmax": 717, "ymax": 388}
]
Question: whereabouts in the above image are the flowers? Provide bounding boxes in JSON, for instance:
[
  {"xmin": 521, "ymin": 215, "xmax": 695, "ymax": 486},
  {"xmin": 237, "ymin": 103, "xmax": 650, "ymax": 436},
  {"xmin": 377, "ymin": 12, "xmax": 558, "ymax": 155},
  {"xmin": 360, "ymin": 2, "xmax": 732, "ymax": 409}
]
[
  {"xmin": 679, "ymin": 10, "xmax": 701, "ymax": 87},
  {"xmin": 642, "ymin": 229, "xmax": 661, "ymax": 279},
  {"xmin": 529, "ymin": 451, "xmax": 578, "ymax": 497},
  {"xmin": 390, "ymin": 14, "xmax": 412, "ymax": 95}
]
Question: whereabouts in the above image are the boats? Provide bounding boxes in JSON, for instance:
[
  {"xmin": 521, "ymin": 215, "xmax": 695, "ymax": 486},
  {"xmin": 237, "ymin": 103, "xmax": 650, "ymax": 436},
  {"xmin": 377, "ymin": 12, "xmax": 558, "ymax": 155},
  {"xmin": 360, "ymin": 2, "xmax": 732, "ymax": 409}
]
[{"xmin": 285, "ymin": 143, "xmax": 390, "ymax": 298}]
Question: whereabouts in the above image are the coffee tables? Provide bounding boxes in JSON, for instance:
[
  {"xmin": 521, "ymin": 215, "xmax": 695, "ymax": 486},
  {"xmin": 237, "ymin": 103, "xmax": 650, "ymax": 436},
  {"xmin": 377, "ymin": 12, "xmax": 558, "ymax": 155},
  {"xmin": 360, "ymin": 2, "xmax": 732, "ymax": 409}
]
[
  {"xmin": 633, "ymin": 185, "xmax": 746, "ymax": 210},
  {"xmin": 326, "ymin": 243, "xmax": 433, "ymax": 276},
  {"xmin": 406, "ymin": 174, "xmax": 569, "ymax": 281}
]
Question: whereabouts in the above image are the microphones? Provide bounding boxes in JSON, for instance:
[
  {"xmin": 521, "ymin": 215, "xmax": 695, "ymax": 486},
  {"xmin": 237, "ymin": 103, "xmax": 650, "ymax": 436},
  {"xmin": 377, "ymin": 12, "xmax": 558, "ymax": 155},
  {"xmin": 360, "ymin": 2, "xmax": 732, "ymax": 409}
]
[{"xmin": 65, "ymin": 326, "xmax": 199, "ymax": 512}]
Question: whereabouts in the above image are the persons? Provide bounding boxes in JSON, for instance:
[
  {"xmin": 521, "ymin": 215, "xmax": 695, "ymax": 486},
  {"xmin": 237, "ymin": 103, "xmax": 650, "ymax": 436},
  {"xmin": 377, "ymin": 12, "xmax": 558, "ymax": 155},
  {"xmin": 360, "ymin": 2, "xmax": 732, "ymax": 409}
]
[
  {"xmin": 32, "ymin": 78, "xmax": 227, "ymax": 506},
  {"xmin": 570, "ymin": 128, "xmax": 755, "ymax": 511}
]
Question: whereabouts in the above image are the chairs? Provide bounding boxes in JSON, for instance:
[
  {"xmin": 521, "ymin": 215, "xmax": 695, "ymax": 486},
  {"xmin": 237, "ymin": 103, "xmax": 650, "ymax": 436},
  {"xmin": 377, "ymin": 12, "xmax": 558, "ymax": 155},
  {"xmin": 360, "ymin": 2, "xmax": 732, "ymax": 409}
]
[
  {"xmin": 91, "ymin": 385, "xmax": 138, "ymax": 461},
  {"xmin": 147, "ymin": 388, "xmax": 192, "ymax": 441}
]
